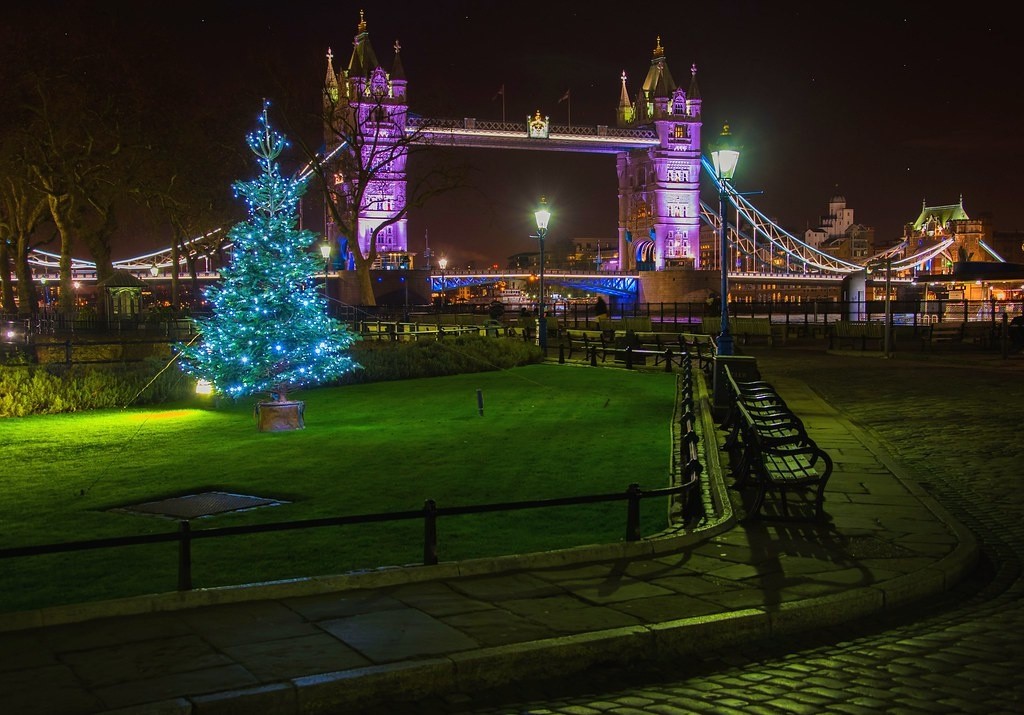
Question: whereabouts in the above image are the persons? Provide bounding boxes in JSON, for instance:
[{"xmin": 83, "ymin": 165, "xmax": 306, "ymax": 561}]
[
  {"xmin": 705, "ymin": 292, "xmax": 720, "ymax": 317},
  {"xmin": 521, "ymin": 308, "xmax": 531, "ymax": 317},
  {"xmin": 532, "ymin": 308, "xmax": 540, "ymax": 318},
  {"xmin": 595, "ymin": 297, "xmax": 607, "ymax": 322}
]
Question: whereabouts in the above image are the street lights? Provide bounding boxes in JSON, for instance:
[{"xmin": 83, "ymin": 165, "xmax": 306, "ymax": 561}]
[
  {"xmin": 708, "ymin": 121, "xmax": 746, "ymax": 359},
  {"xmin": 318, "ymin": 238, "xmax": 335, "ymax": 327},
  {"xmin": 436, "ymin": 251, "xmax": 447, "ymax": 310},
  {"xmin": 531, "ymin": 195, "xmax": 552, "ymax": 357}
]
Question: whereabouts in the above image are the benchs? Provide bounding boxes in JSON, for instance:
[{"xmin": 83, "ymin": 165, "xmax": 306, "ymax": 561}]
[
  {"xmin": 566, "ymin": 315, "xmax": 896, "ymax": 525},
  {"xmin": 921, "ymin": 323, "xmax": 993, "ymax": 353},
  {"xmin": 419, "ymin": 325, "xmax": 529, "ymax": 343}
]
